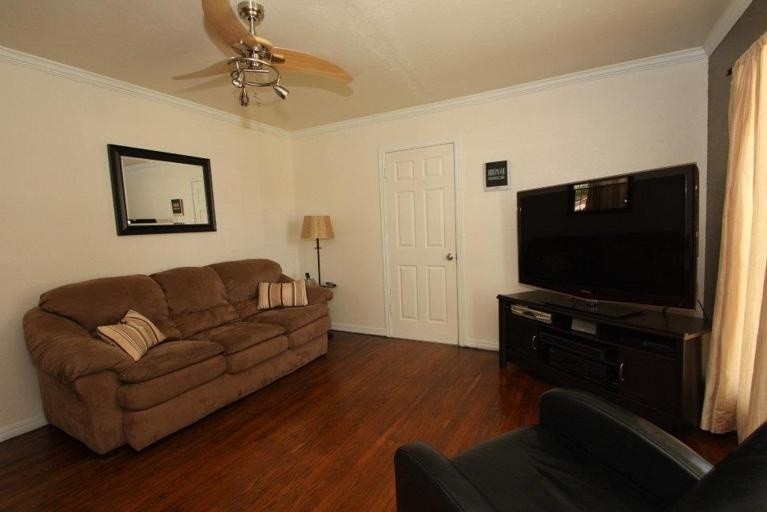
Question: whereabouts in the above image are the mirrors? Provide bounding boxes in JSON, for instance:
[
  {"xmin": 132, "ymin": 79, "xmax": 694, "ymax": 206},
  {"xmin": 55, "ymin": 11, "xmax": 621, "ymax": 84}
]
[{"xmin": 107, "ymin": 143, "xmax": 218, "ymax": 236}]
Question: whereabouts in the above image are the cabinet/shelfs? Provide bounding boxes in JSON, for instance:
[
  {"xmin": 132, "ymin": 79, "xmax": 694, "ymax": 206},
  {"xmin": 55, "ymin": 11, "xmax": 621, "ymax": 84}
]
[{"xmin": 496, "ymin": 290, "xmax": 713, "ymax": 437}]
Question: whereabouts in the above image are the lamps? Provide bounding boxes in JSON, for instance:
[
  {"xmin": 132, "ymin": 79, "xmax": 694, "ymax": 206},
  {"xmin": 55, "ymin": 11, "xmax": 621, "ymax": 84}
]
[
  {"xmin": 173, "ymin": 0, "xmax": 352, "ymax": 106},
  {"xmin": 300, "ymin": 215, "xmax": 334, "ymax": 285}
]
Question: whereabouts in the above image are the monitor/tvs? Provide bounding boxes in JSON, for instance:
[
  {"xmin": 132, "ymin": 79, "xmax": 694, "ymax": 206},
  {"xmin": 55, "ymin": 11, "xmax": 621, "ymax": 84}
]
[{"xmin": 516, "ymin": 162, "xmax": 699, "ymax": 320}]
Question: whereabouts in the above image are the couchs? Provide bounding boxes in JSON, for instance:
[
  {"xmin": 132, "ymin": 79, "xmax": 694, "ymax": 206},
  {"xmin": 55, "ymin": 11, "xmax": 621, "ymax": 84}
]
[
  {"xmin": 394, "ymin": 389, "xmax": 767, "ymax": 512},
  {"xmin": 22, "ymin": 258, "xmax": 334, "ymax": 454}
]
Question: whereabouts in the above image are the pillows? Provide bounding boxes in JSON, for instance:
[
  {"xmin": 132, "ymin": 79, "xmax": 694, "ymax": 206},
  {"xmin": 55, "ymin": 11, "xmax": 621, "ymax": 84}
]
[
  {"xmin": 96, "ymin": 310, "xmax": 167, "ymax": 362},
  {"xmin": 257, "ymin": 280, "xmax": 308, "ymax": 310}
]
